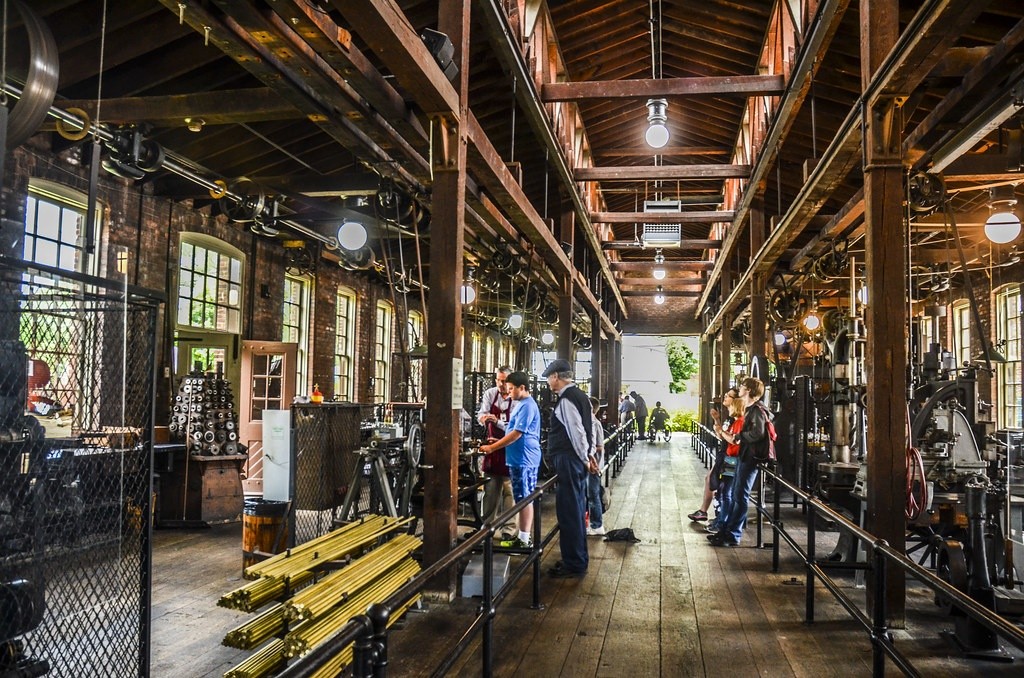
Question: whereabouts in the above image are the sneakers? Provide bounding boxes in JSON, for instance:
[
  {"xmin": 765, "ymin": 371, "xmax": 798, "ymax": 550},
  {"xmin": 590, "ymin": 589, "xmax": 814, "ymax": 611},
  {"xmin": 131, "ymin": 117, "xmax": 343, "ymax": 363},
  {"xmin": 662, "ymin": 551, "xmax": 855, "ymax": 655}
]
[{"xmin": 688, "ymin": 510, "xmax": 708, "ymax": 520}]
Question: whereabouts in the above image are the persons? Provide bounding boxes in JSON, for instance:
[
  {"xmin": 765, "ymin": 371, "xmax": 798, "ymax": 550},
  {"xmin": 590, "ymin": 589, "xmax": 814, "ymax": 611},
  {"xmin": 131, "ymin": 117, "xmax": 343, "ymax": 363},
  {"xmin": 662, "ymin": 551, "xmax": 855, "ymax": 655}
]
[
  {"xmin": 479, "ymin": 372, "xmax": 542, "ymax": 556},
  {"xmin": 585, "ymin": 397, "xmax": 605, "ymax": 536},
  {"xmin": 688, "ymin": 378, "xmax": 777, "ymax": 546},
  {"xmin": 629, "ymin": 391, "xmax": 648, "ymax": 438},
  {"xmin": 649, "ymin": 401, "xmax": 671, "ymax": 428},
  {"xmin": 619, "ymin": 391, "xmax": 635, "ymax": 422},
  {"xmin": 464, "ymin": 365, "xmax": 518, "ymax": 541},
  {"xmin": 542, "ymin": 359, "xmax": 600, "ymax": 577}
]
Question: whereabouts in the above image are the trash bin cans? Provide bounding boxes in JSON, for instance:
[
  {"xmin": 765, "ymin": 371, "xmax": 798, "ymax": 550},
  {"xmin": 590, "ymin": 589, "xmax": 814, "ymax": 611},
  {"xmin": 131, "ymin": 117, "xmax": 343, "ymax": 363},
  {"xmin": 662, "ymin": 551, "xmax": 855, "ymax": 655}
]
[{"xmin": 241, "ymin": 498, "xmax": 288, "ymax": 582}]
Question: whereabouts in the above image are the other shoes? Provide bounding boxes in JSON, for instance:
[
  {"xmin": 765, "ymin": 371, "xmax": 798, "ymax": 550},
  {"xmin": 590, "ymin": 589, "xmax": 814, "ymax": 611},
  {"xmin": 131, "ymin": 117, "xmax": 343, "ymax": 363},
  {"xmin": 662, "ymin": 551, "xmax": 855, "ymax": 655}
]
[
  {"xmin": 698, "ymin": 523, "xmax": 720, "ymax": 533},
  {"xmin": 465, "ymin": 530, "xmax": 478, "ymax": 538},
  {"xmin": 500, "ymin": 535, "xmax": 534, "ymax": 548},
  {"xmin": 711, "ymin": 536, "xmax": 740, "ymax": 547},
  {"xmin": 586, "ymin": 524, "xmax": 605, "ymax": 536},
  {"xmin": 547, "ymin": 565, "xmax": 580, "ymax": 578}
]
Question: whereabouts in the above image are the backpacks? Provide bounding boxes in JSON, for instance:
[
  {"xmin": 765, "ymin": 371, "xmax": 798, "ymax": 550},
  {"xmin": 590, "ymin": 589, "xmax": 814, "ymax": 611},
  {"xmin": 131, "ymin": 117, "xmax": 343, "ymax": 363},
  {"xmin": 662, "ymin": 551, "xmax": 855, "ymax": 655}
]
[{"xmin": 760, "ymin": 406, "xmax": 778, "ymax": 462}]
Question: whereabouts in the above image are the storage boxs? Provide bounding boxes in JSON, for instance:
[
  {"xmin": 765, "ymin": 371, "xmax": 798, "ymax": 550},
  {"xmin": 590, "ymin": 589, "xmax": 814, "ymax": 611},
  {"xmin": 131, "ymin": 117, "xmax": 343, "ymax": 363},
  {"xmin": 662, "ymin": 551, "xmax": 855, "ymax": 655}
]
[{"xmin": 83, "ymin": 431, "xmax": 135, "ymax": 448}]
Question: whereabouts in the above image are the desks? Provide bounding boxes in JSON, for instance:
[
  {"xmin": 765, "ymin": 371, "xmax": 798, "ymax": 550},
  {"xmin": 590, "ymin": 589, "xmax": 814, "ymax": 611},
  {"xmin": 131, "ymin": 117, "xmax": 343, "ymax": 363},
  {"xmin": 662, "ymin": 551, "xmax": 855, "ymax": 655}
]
[{"xmin": 52, "ymin": 442, "xmax": 189, "ymax": 540}]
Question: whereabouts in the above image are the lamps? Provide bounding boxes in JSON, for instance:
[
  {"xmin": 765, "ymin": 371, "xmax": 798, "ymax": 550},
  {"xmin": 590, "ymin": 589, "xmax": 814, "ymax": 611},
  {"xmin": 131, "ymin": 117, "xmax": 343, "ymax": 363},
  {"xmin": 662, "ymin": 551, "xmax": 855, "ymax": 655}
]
[
  {"xmin": 541, "ymin": 324, "xmax": 554, "ymax": 344},
  {"xmin": 653, "ymin": 248, "xmax": 665, "ymax": 279},
  {"xmin": 857, "ymin": 286, "xmax": 867, "ymax": 305},
  {"xmin": 804, "ymin": 299, "xmax": 819, "ymax": 330},
  {"xmin": 645, "ymin": 98, "xmax": 669, "ymax": 148},
  {"xmin": 338, "ymin": 222, "xmax": 367, "ymax": 250},
  {"xmin": 509, "ymin": 314, "xmax": 523, "ymax": 328},
  {"xmin": 655, "ymin": 285, "xmax": 665, "ymax": 304},
  {"xmin": 985, "ymin": 184, "xmax": 1021, "ymax": 243},
  {"xmin": 775, "ymin": 325, "xmax": 786, "ymax": 345},
  {"xmin": 460, "ymin": 267, "xmax": 476, "ymax": 304}
]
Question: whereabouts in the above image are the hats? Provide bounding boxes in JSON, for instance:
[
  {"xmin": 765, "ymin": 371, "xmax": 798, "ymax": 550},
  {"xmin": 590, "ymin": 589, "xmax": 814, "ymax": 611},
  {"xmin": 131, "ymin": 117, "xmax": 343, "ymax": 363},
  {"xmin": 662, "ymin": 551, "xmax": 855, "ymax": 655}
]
[{"xmin": 542, "ymin": 360, "xmax": 571, "ymax": 377}]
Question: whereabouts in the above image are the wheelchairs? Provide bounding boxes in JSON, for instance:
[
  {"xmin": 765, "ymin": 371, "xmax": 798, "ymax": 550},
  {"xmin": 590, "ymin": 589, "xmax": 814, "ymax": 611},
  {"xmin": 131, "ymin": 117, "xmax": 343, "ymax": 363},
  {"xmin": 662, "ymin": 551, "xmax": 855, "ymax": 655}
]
[{"xmin": 646, "ymin": 419, "xmax": 672, "ymax": 442}]
